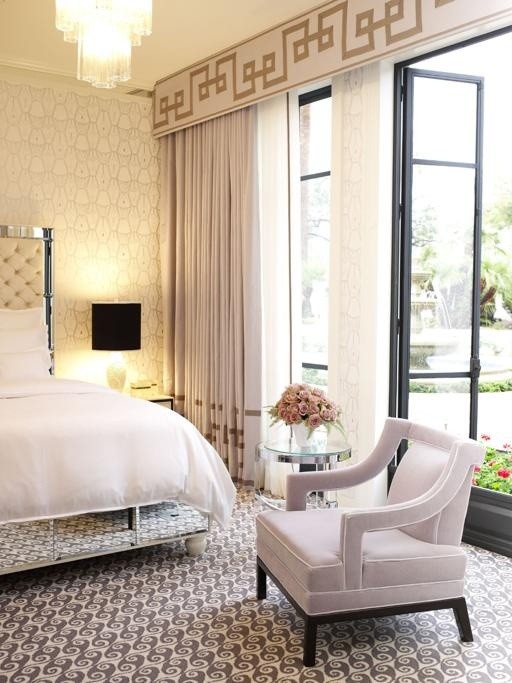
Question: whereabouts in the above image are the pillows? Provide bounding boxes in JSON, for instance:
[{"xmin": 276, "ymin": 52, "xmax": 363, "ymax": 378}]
[{"xmin": 0, "ymin": 308, "xmax": 54, "ymax": 398}]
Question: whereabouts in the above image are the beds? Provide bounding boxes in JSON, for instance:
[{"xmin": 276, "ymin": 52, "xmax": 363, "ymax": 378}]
[{"xmin": 0, "ymin": 220, "xmax": 238, "ymax": 589}]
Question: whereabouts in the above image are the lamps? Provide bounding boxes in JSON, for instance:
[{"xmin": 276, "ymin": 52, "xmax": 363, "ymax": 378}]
[
  {"xmin": 54, "ymin": 0, "xmax": 156, "ymax": 90},
  {"xmin": 90, "ymin": 298, "xmax": 143, "ymax": 391}
]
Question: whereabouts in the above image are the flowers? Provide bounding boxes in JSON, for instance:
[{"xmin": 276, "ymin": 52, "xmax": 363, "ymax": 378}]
[{"xmin": 263, "ymin": 381, "xmax": 349, "ymax": 443}]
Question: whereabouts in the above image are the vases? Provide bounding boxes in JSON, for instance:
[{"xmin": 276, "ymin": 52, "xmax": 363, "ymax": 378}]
[{"xmin": 291, "ymin": 420, "xmax": 317, "ymax": 448}]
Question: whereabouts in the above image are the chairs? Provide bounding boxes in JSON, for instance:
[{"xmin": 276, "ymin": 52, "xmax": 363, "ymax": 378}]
[{"xmin": 254, "ymin": 412, "xmax": 486, "ymax": 667}]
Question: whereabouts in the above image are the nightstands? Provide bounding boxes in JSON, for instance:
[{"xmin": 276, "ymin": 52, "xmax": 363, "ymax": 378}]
[{"xmin": 131, "ymin": 391, "xmax": 176, "ymax": 413}]
